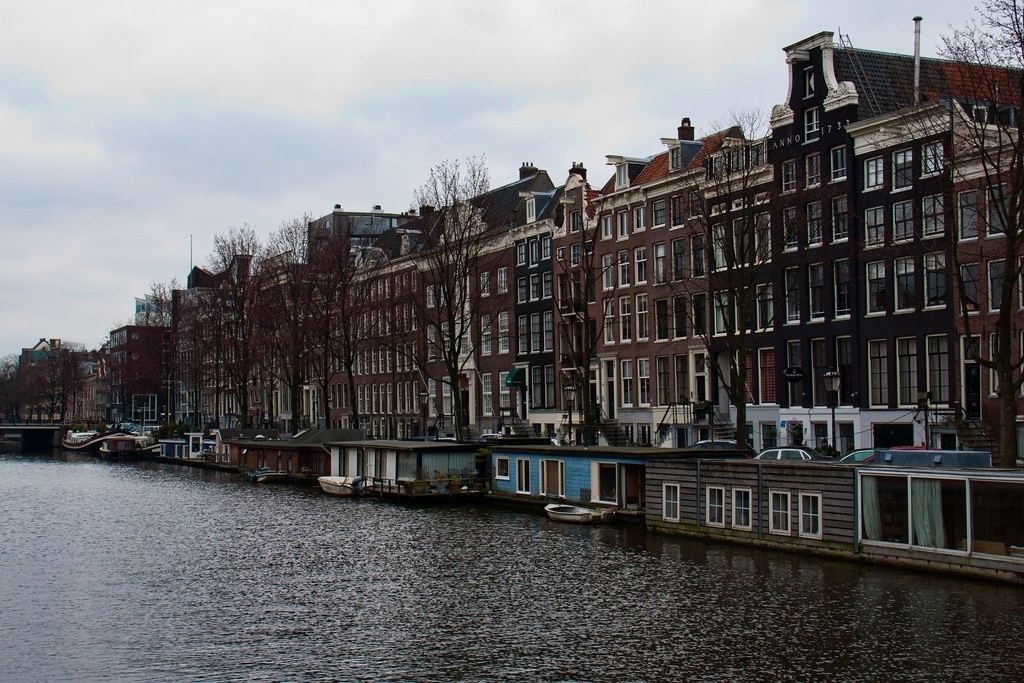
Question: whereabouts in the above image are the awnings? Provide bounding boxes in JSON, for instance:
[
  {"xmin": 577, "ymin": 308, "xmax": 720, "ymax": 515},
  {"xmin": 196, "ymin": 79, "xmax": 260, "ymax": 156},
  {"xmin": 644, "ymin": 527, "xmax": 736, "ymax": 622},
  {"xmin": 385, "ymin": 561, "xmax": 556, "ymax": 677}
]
[{"xmin": 506, "ymin": 368, "xmax": 525, "ymax": 386}]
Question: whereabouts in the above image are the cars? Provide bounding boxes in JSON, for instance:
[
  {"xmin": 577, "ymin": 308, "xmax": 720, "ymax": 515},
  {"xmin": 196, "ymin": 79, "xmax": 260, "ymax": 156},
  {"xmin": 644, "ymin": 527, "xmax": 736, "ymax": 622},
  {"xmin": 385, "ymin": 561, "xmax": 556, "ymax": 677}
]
[
  {"xmin": 434, "ymin": 437, "xmax": 457, "ymax": 442},
  {"xmin": 538, "ymin": 436, "xmax": 569, "ymax": 446},
  {"xmin": 479, "ymin": 433, "xmax": 504, "ymax": 439},
  {"xmin": 685, "ymin": 442, "xmax": 757, "ymax": 460},
  {"xmin": 752, "ymin": 445, "xmax": 834, "ymax": 460},
  {"xmin": 833, "ymin": 448, "xmax": 875, "ymax": 463},
  {"xmin": 397, "ymin": 436, "xmax": 434, "ymax": 441},
  {"xmin": 118, "ymin": 422, "xmax": 160, "ymax": 435}
]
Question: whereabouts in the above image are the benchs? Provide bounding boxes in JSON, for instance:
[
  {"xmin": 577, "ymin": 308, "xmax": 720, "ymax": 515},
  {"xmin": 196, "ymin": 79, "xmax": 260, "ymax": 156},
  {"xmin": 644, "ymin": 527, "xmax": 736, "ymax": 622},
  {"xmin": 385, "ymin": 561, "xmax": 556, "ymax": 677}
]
[{"xmin": 959, "ymin": 538, "xmax": 1007, "ymax": 556}]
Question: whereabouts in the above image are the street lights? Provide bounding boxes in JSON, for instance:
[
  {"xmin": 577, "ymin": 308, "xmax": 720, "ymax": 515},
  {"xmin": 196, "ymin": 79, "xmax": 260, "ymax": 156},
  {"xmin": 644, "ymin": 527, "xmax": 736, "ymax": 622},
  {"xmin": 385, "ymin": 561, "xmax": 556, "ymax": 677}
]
[
  {"xmin": 327, "ymin": 396, "xmax": 332, "ymax": 429},
  {"xmin": 822, "ymin": 366, "xmax": 842, "ymax": 458},
  {"xmin": 562, "ymin": 383, "xmax": 576, "ymax": 440},
  {"xmin": 312, "ymin": 396, "xmax": 316, "ymax": 423},
  {"xmin": 418, "ymin": 390, "xmax": 429, "ymax": 435},
  {"xmin": 112, "ymin": 407, "xmax": 117, "ymax": 429}
]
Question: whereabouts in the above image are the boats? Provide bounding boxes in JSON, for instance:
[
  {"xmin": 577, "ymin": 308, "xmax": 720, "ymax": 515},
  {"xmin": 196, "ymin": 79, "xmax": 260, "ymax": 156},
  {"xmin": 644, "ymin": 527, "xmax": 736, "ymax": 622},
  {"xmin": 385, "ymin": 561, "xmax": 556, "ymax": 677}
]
[
  {"xmin": 99, "ymin": 435, "xmax": 161, "ymax": 461},
  {"xmin": 62, "ymin": 429, "xmax": 135, "ymax": 452},
  {"xmin": 317, "ymin": 475, "xmax": 363, "ymax": 497},
  {"xmin": 245, "ymin": 467, "xmax": 288, "ymax": 483},
  {"xmin": 544, "ymin": 503, "xmax": 608, "ymax": 523}
]
